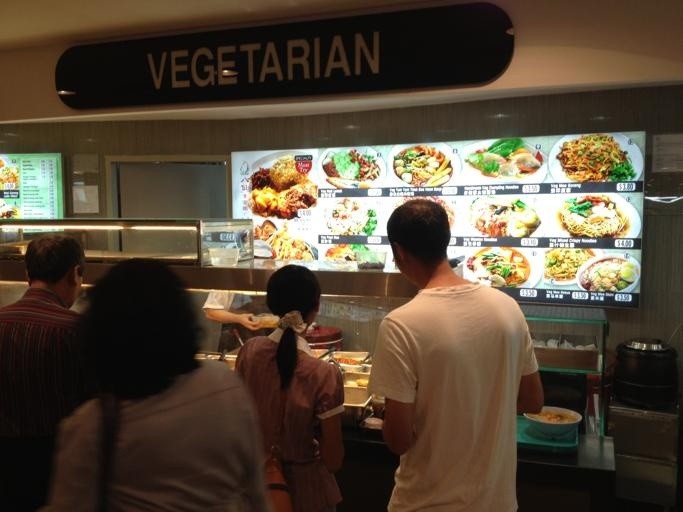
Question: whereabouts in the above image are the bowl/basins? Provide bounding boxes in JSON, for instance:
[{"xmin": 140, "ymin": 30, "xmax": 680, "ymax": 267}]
[
  {"xmin": 576, "ymin": 253, "xmax": 643, "ymax": 294},
  {"xmin": 257, "ymin": 219, "xmax": 279, "ymax": 242},
  {"xmin": 522, "ymin": 405, "xmax": 583, "ymax": 440}
]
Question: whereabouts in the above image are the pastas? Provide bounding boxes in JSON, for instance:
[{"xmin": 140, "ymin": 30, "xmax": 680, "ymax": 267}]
[
  {"xmin": 545, "ymin": 249, "xmax": 595, "ymax": 281},
  {"xmin": 556, "ymin": 135, "xmax": 626, "ymax": 182},
  {"xmin": 562, "ymin": 209, "xmax": 627, "ymax": 239}
]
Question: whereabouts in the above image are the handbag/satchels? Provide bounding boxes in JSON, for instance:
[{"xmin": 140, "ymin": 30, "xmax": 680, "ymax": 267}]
[{"xmin": 256, "ymin": 451, "xmax": 293, "ymax": 511}]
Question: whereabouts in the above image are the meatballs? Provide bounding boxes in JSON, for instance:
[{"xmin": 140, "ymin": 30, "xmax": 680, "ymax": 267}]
[
  {"xmin": 401, "ymin": 173, "xmax": 413, "ymax": 182},
  {"xmin": 396, "ymin": 166, "xmax": 406, "ymax": 175},
  {"xmin": 395, "ymin": 158, "xmax": 404, "ymax": 166}
]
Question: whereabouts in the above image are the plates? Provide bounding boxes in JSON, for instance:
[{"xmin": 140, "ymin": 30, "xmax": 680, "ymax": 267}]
[
  {"xmin": 459, "ymin": 137, "xmax": 548, "ymax": 186},
  {"xmin": 550, "ymin": 191, "xmax": 642, "ymax": 239},
  {"xmin": 266, "ymin": 237, "xmax": 319, "ymax": 261},
  {"xmin": 318, "ymin": 146, "xmax": 387, "ymax": 188},
  {"xmin": 388, "ymin": 142, "xmax": 463, "ymax": 187},
  {"xmin": 246, "ymin": 150, "xmax": 322, "ymax": 219},
  {"xmin": 540, "ymin": 248, "xmax": 603, "ymax": 285},
  {"xmin": 462, "ymin": 245, "xmax": 542, "ymax": 289},
  {"xmin": 467, "ymin": 195, "xmax": 550, "ymax": 237},
  {"xmin": 321, "ymin": 195, "xmax": 393, "ymax": 236},
  {"xmin": 548, "ymin": 133, "xmax": 644, "ymax": 181}
]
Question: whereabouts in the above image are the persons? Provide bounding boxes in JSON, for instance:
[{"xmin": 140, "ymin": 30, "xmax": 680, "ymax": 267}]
[
  {"xmin": 238, "ymin": 263, "xmax": 346, "ymax": 512},
  {"xmin": 41, "ymin": 253, "xmax": 274, "ymax": 512},
  {"xmin": 0, "ymin": 233, "xmax": 91, "ymax": 512},
  {"xmin": 370, "ymin": 199, "xmax": 546, "ymax": 512},
  {"xmin": 200, "ymin": 239, "xmax": 278, "ymax": 355}
]
